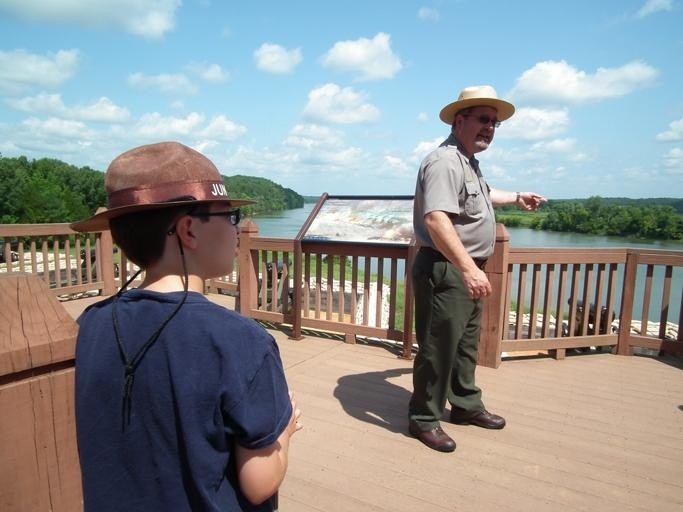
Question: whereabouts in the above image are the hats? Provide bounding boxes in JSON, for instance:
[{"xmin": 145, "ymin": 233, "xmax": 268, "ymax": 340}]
[
  {"xmin": 69, "ymin": 142, "xmax": 258, "ymax": 232},
  {"xmin": 439, "ymin": 86, "xmax": 515, "ymax": 125}
]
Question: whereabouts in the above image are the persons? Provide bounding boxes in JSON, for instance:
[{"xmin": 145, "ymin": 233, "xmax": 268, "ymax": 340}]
[
  {"xmin": 408, "ymin": 84, "xmax": 547, "ymax": 451},
  {"xmin": 70, "ymin": 141, "xmax": 303, "ymax": 512}
]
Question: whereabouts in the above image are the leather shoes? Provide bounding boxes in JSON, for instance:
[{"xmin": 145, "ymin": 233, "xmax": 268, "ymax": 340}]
[
  {"xmin": 409, "ymin": 424, "xmax": 456, "ymax": 453},
  {"xmin": 451, "ymin": 410, "xmax": 506, "ymax": 429}
]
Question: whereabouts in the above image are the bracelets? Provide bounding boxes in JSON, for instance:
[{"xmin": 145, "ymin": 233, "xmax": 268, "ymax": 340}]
[{"xmin": 516, "ymin": 192, "xmax": 519, "ymax": 203}]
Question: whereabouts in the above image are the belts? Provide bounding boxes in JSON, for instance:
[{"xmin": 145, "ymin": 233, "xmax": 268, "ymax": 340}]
[{"xmin": 473, "ymin": 258, "xmax": 488, "ymax": 270}]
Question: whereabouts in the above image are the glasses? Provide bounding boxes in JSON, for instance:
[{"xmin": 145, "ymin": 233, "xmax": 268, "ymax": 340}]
[
  {"xmin": 463, "ymin": 113, "xmax": 500, "ymax": 128},
  {"xmin": 168, "ymin": 209, "xmax": 240, "ymax": 236}
]
